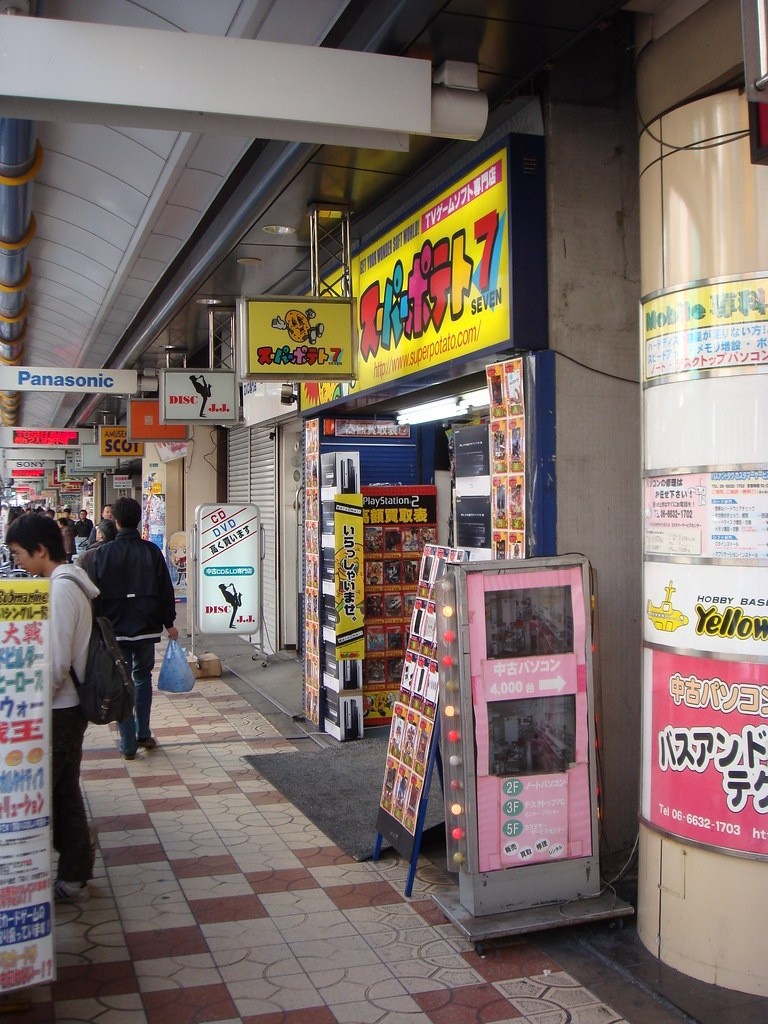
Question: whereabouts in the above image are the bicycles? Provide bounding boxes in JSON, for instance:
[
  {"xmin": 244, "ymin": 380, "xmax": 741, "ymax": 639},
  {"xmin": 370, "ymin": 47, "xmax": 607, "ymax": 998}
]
[{"xmin": 0, "ymin": 561, "xmax": 32, "ymax": 578}]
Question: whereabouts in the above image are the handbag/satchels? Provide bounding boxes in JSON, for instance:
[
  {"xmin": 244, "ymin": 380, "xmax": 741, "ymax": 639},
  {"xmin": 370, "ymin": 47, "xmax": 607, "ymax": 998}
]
[{"xmin": 157, "ymin": 639, "xmax": 195, "ymax": 693}]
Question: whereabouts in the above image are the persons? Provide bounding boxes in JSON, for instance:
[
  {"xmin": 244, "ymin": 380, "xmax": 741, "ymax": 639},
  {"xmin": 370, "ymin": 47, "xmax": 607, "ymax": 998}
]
[
  {"xmin": 5, "ymin": 515, "xmax": 100, "ymax": 900},
  {"xmin": 57, "ymin": 518, "xmax": 75, "ymax": 564},
  {"xmin": 529, "ymin": 615, "xmax": 539, "ymax": 650},
  {"xmin": 88, "ymin": 504, "xmax": 118, "ymax": 545},
  {"xmin": 0, "ymin": 502, "xmax": 54, "ymax": 521},
  {"xmin": 74, "ymin": 510, "xmax": 93, "ymax": 555},
  {"xmin": 91, "ymin": 496, "xmax": 178, "ymax": 760},
  {"xmin": 75, "ymin": 519, "xmax": 119, "ymax": 584},
  {"xmin": 530, "ymin": 734, "xmax": 544, "ymax": 770},
  {"xmin": 62, "ymin": 508, "xmax": 74, "ymax": 525}
]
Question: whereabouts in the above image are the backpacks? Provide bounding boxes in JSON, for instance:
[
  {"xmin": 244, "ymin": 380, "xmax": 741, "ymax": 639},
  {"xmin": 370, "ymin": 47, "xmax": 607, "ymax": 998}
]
[{"xmin": 58, "ymin": 573, "xmax": 135, "ymax": 726}]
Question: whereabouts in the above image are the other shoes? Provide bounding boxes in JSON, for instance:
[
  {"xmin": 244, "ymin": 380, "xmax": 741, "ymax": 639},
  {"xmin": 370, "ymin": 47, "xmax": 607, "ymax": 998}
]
[
  {"xmin": 124, "ymin": 753, "xmax": 136, "ymax": 760},
  {"xmin": 137, "ymin": 738, "xmax": 157, "ymax": 748},
  {"xmin": 54, "ymin": 879, "xmax": 89, "ymax": 903}
]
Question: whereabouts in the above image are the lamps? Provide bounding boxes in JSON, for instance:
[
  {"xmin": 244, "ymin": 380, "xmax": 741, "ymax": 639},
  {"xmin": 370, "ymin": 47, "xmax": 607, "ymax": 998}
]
[{"xmin": 281, "ymin": 384, "xmax": 299, "ymax": 406}]
